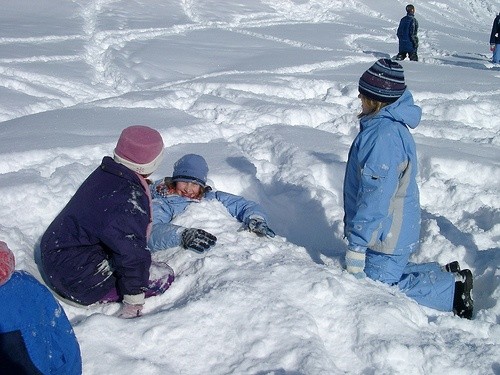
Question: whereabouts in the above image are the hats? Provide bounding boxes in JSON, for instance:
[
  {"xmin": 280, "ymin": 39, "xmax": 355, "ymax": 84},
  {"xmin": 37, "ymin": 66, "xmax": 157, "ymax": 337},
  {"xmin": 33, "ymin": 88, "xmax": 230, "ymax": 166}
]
[
  {"xmin": 113, "ymin": 125, "xmax": 165, "ymax": 174},
  {"xmin": 172, "ymin": 154, "xmax": 209, "ymax": 188},
  {"xmin": 0, "ymin": 241, "xmax": 15, "ymax": 285},
  {"xmin": 358, "ymin": 59, "xmax": 405, "ymax": 102}
]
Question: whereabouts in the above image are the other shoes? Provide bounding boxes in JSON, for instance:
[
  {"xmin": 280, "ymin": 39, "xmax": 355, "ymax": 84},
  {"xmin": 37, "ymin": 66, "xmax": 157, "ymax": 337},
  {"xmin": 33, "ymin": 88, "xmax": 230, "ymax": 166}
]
[
  {"xmin": 444, "ymin": 261, "xmax": 460, "ymax": 273},
  {"xmin": 453, "ymin": 269, "xmax": 473, "ymax": 320}
]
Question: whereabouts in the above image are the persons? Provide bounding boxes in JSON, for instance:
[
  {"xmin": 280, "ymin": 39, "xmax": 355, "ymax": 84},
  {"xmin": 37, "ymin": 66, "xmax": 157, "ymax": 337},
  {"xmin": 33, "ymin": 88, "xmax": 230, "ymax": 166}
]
[
  {"xmin": 147, "ymin": 154, "xmax": 275, "ymax": 253},
  {"xmin": 33, "ymin": 124, "xmax": 174, "ymax": 318},
  {"xmin": 489, "ymin": 13, "xmax": 500, "ymax": 62},
  {"xmin": 0, "ymin": 240, "xmax": 82, "ymax": 375},
  {"xmin": 392, "ymin": 5, "xmax": 419, "ymax": 61},
  {"xmin": 344, "ymin": 57, "xmax": 475, "ymax": 322}
]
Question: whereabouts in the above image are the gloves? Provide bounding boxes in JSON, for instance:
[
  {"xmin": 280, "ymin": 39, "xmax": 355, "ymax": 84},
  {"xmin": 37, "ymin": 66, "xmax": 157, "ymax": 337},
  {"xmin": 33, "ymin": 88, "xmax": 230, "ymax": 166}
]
[
  {"xmin": 346, "ymin": 249, "xmax": 367, "ymax": 280},
  {"xmin": 181, "ymin": 228, "xmax": 217, "ymax": 254},
  {"xmin": 490, "ymin": 43, "xmax": 496, "ymax": 51},
  {"xmin": 244, "ymin": 218, "xmax": 277, "ymax": 239},
  {"xmin": 121, "ymin": 292, "xmax": 145, "ymax": 318}
]
